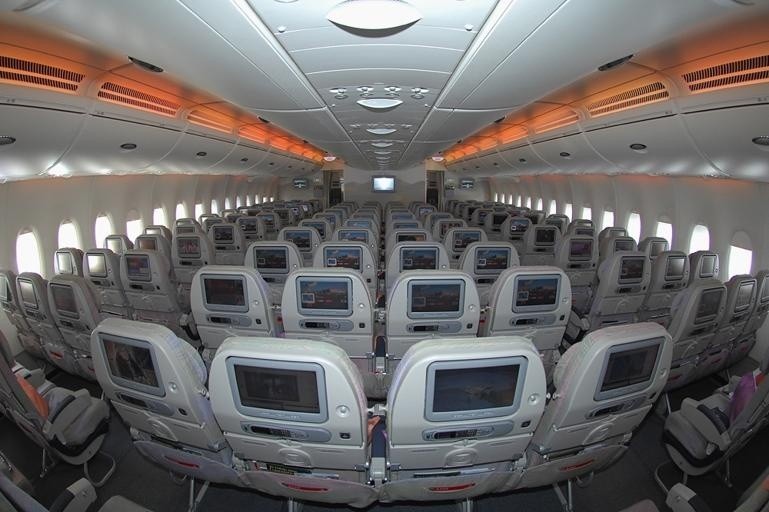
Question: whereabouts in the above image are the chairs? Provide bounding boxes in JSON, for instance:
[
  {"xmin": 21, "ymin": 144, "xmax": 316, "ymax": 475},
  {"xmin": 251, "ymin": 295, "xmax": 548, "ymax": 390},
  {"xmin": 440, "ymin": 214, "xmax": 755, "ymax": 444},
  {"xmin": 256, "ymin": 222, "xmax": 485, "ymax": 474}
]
[{"xmin": 2, "ymin": 197, "xmax": 768, "ymax": 512}]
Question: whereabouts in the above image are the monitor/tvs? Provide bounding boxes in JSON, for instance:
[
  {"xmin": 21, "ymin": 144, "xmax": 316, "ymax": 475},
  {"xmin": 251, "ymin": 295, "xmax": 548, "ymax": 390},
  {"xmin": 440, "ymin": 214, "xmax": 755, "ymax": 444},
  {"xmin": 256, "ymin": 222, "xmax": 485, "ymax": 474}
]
[
  {"xmin": 424, "ymin": 356, "xmax": 528, "ymax": 422},
  {"xmin": 97, "ymin": 333, "xmax": 165, "ymax": 396},
  {"xmin": 17, "ymin": 278, "xmax": 38, "ymax": 309},
  {"xmin": 226, "ymin": 357, "xmax": 328, "ymax": 424},
  {"xmin": 296, "ymin": 277, "xmax": 353, "ymax": 317},
  {"xmin": 512, "ymin": 275, "xmax": 560, "ymax": 312},
  {"xmin": 50, "ymin": 284, "xmax": 80, "ymax": 319},
  {"xmin": 695, "ymin": 287, "xmax": 724, "ymax": 324},
  {"xmin": 736, "ymin": 282, "xmax": 754, "ymax": 312},
  {"xmin": 199, "ymin": 274, "xmax": 249, "ymax": 313},
  {"xmin": 407, "ymin": 280, "xmax": 465, "ymax": 319},
  {"xmin": 1, "ymin": 276, "xmax": 8, "ymax": 302},
  {"xmin": 594, "ymin": 337, "xmax": 666, "ymax": 400},
  {"xmin": 371, "ymin": 175, "xmax": 396, "ymax": 192},
  {"xmin": 761, "ymin": 276, "xmax": 769, "ymax": 302},
  {"xmin": 0, "ymin": 200, "xmax": 769, "ymax": 284}
]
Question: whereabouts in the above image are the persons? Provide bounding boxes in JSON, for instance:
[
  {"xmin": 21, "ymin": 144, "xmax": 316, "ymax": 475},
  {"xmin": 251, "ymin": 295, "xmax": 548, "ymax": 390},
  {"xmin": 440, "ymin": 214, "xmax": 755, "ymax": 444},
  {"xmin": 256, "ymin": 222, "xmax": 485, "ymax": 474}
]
[{"xmin": 115, "ymin": 341, "xmax": 151, "ymax": 386}]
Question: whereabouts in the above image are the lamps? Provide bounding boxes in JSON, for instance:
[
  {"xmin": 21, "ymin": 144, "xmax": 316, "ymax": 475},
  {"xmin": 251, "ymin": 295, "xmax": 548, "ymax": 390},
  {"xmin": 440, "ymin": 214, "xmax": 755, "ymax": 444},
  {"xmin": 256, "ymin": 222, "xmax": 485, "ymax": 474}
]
[
  {"xmin": 324, "ymin": 0, "xmax": 423, "ymax": 31},
  {"xmin": 357, "ymin": 99, "xmax": 404, "ymax": 108}
]
